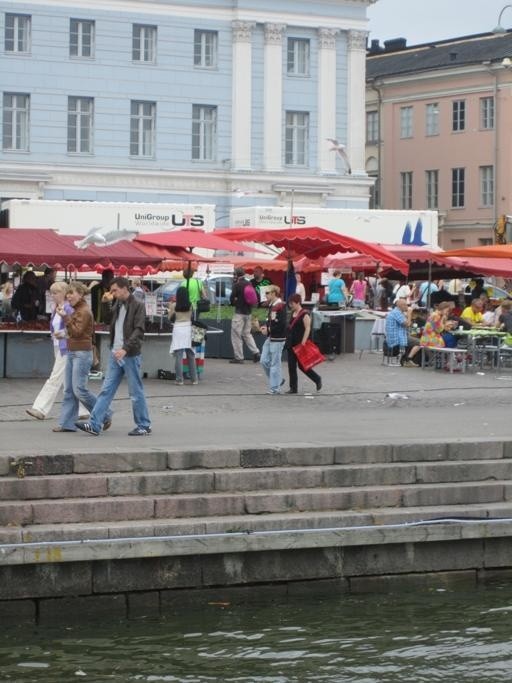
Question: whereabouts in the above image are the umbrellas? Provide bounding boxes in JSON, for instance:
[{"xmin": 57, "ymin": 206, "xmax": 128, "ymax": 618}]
[
  {"xmin": 428, "ymin": 243, "xmax": 512, "ymax": 261},
  {"xmin": 211, "ymin": 226, "xmax": 409, "ymax": 305},
  {"xmin": 133, "ymin": 227, "xmax": 273, "ymax": 270}
]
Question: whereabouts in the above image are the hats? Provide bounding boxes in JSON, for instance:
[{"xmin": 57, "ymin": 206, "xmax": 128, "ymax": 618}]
[{"xmin": 235, "ymin": 266, "xmax": 245, "ymax": 276}]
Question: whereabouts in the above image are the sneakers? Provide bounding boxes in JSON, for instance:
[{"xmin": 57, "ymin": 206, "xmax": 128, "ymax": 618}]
[
  {"xmin": 27, "ymin": 409, "xmax": 152, "ymax": 435},
  {"xmin": 403, "ymin": 359, "xmax": 420, "ymax": 368},
  {"xmin": 229, "ymin": 353, "xmax": 323, "ymax": 394}
]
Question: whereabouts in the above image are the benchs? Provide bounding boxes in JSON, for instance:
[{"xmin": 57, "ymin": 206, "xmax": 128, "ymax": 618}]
[{"xmin": 419, "ymin": 346, "xmax": 468, "ymax": 374}]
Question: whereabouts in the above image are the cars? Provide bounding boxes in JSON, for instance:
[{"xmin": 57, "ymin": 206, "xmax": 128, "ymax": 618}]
[{"xmin": 149, "ymin": 271, "xmax": 246, "ymax": 324}]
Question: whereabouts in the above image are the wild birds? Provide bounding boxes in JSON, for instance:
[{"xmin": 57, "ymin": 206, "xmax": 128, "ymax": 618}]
[{"xmin": 385, "ymin": 391, "xmax": 409, "ymax": 406}]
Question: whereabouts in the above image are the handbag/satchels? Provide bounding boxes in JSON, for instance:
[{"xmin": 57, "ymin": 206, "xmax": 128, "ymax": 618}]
[
  {"xmin": 197, "ymin": 300, "xmax": 210, "ymax": 312},
  {"xmin": 292, "ymin": 340, "xmax": 327, "ymax": 373},
  {"xmin": 243, "ymin": 284, "xmax": 258, "ymax": 305}
]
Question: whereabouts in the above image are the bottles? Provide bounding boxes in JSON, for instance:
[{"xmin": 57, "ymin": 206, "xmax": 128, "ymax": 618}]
[
  {"xmin": 108, "ymin": 345, "xmax": 126, "ymax": 366},
  {"xmin": 458, "ymin": 321, "xmax": 464, "ymax": 332},
  {"xmin": 16, "ymin": 310, "xmax": 21, "ymax": 329}
]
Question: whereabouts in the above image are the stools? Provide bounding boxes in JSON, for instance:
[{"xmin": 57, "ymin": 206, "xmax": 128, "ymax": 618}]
[{"xmin": 380, "ymin": 336, "xmax": 405, "ymax": 366}]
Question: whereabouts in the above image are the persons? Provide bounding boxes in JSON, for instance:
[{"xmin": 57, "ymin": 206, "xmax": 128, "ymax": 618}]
[
  {"xmin": 168, "ymin": 286, "xmax": 198, "ymax": 385},
  {"xmin": 230, "ymin": 265, "xmax": 321, "ymax": 394},
  {"xmin": 328, "ymin": 271, "xmax": 512, "ymax": 367},
  {"xmin": 1, "ymin": 269, "xmax": 152, "ymax": 436},
  {"xmin": 181, "ymin": 268, "xmax": 205, "ymax": 321}
]
